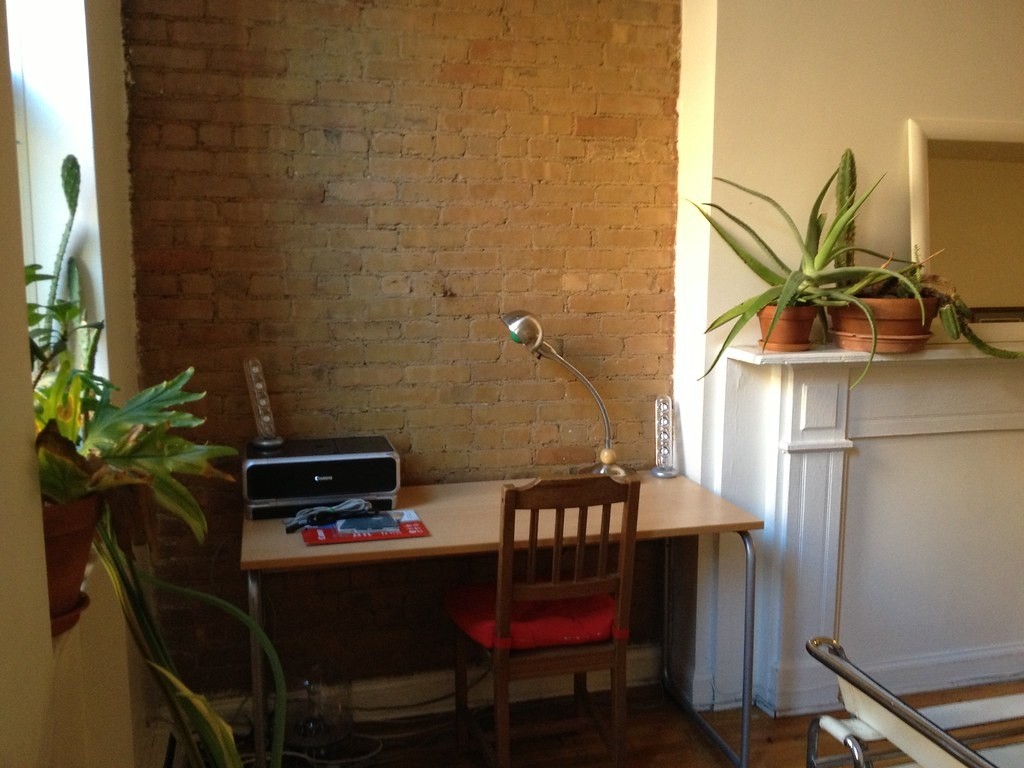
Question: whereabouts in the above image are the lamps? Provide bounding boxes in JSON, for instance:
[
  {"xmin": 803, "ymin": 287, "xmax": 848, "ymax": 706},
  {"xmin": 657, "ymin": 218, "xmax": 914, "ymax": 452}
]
[{"xmin": 500, "ymin": 310, "xmax": 635, "ymax": 477}]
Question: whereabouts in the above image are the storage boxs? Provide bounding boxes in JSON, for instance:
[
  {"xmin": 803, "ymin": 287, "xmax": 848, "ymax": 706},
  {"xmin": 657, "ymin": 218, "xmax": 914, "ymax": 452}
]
[{"xmin": 242, "ymin": 430, "xmax": 400, "ymax": 522}]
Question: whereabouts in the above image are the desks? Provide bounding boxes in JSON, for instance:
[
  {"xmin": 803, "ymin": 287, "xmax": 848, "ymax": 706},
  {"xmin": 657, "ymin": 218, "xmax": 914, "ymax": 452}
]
[{"xmin": 240, "ymin": 471, "xmax": 763, "ymax": 768}]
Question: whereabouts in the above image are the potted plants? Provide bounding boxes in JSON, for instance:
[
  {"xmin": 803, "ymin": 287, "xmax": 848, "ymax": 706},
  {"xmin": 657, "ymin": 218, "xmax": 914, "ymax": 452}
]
[
  {"xmin": 688, "ymin": 171, "xmax": 925, "ymax": 392},
  {"xmin": 24, "ymin": 154, "xmax": 243, "ymax": 636},
  {"xmin": 827, "ymin": 148, "xmax": 1024, "ymax": 360}
]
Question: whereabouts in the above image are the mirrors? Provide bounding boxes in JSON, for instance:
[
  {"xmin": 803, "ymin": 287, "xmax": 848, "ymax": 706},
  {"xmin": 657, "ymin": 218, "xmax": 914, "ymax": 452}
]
[{"xmin": 898, "ymin": 116, "xmax": 1024, "ymax": 342}]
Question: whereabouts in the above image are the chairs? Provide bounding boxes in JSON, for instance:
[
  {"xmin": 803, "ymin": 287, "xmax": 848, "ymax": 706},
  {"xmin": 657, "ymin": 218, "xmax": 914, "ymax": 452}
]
[{"xmin": 449, "ymin": 477, "xmax": 641, "ymax": 768}]
